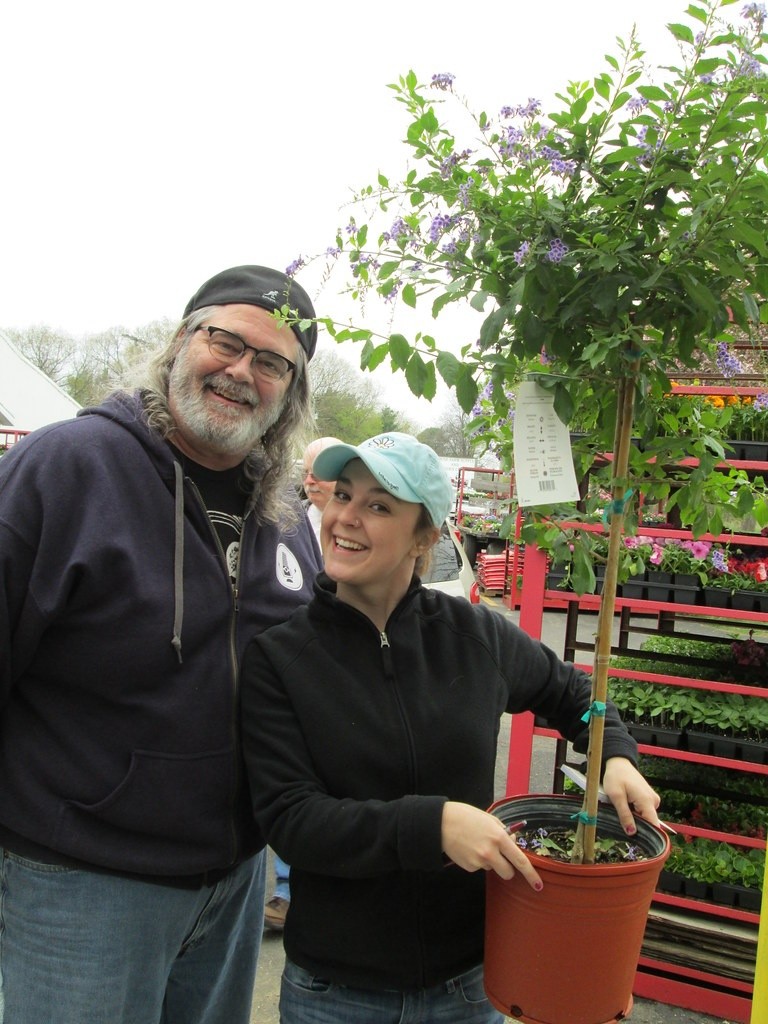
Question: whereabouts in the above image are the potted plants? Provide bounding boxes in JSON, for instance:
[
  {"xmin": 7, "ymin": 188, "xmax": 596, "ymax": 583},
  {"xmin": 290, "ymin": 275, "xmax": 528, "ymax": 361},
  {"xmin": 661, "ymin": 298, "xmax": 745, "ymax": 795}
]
[{"xmin": 583, "ymin": 635, "xmax": 767, "ymax": 914}]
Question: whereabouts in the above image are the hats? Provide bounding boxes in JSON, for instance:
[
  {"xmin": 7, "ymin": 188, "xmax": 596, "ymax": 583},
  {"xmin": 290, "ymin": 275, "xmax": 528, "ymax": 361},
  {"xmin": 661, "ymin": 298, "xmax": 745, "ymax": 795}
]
[
  {"xmin": 312, "ymin": 431, "xmax": 452, "ymax": 531},
  {"xmin": 182, "ymin": 265, "xmax": 317, "ymax": 363}
]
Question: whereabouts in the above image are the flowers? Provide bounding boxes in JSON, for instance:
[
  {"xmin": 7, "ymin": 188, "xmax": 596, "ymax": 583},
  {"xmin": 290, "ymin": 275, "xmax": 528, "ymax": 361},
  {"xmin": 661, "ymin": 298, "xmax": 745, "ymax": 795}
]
[
  {"xmin": 732, "ymin": 626, "xmax": 766, "ymax": 665},
  {"xmin": 656, "ymin": 382, "xmax": 768, "ymax": 440},
  {"xmin": 265, "ymin": 0, "xmax": 768, "ymax": 859},
  {"xmin": 568, "ymin": 532, "xmax": 768, "ymax": 590}
]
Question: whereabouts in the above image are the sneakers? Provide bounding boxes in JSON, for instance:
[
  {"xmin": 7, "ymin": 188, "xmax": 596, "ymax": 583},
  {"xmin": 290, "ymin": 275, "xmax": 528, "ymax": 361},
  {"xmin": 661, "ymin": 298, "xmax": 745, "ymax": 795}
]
[{"xmin": 262, "ymin": 897, "xmax": 290, "ymax": 930}]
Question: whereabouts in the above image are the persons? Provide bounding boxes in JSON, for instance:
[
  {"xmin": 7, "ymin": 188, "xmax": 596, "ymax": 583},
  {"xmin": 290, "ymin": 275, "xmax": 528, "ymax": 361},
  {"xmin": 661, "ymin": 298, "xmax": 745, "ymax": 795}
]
[
  {"xmin": 0, "ymin": 261, "xmax": 320, "ymax": 1024},
  {"xmin": 256, "ymin": 435, "xmax": 356, "ymax": 937},
  {"xmin": 238, "ymin": 431, "xmax": 664, "ymax": 1023}
]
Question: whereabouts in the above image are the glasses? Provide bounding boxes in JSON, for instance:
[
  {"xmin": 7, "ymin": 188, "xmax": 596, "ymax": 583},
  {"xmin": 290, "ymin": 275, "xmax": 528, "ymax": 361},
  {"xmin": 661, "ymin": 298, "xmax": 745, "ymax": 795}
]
[
  {"xmin": 301, "ymin": 471, "xmax": 322, "ymax": 482},
  {"xmin": 195, "ymin": 324, "xmax": 298, "ymax": 383}
]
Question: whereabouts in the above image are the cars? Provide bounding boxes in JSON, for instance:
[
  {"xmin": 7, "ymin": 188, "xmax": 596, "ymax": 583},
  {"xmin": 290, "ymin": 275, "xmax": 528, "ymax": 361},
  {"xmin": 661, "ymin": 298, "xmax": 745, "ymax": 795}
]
[{"xmin": 416, "ymin": 520, "xmax": 481, "ymax": 606}]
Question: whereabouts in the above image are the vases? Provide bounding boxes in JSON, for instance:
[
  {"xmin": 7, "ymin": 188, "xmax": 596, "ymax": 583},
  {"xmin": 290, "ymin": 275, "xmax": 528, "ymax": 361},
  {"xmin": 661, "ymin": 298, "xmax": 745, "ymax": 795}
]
[
  {"xmin": 476, "ymin": 789, "xmax": 668, "ymax": 1024},
  {"xmin": 568, "ymin": 430, "xmax": 767, "ymax": 460},
  {"xmin": 545, "ymin": 559, "xmax": 768, "ymax": 612}
]
